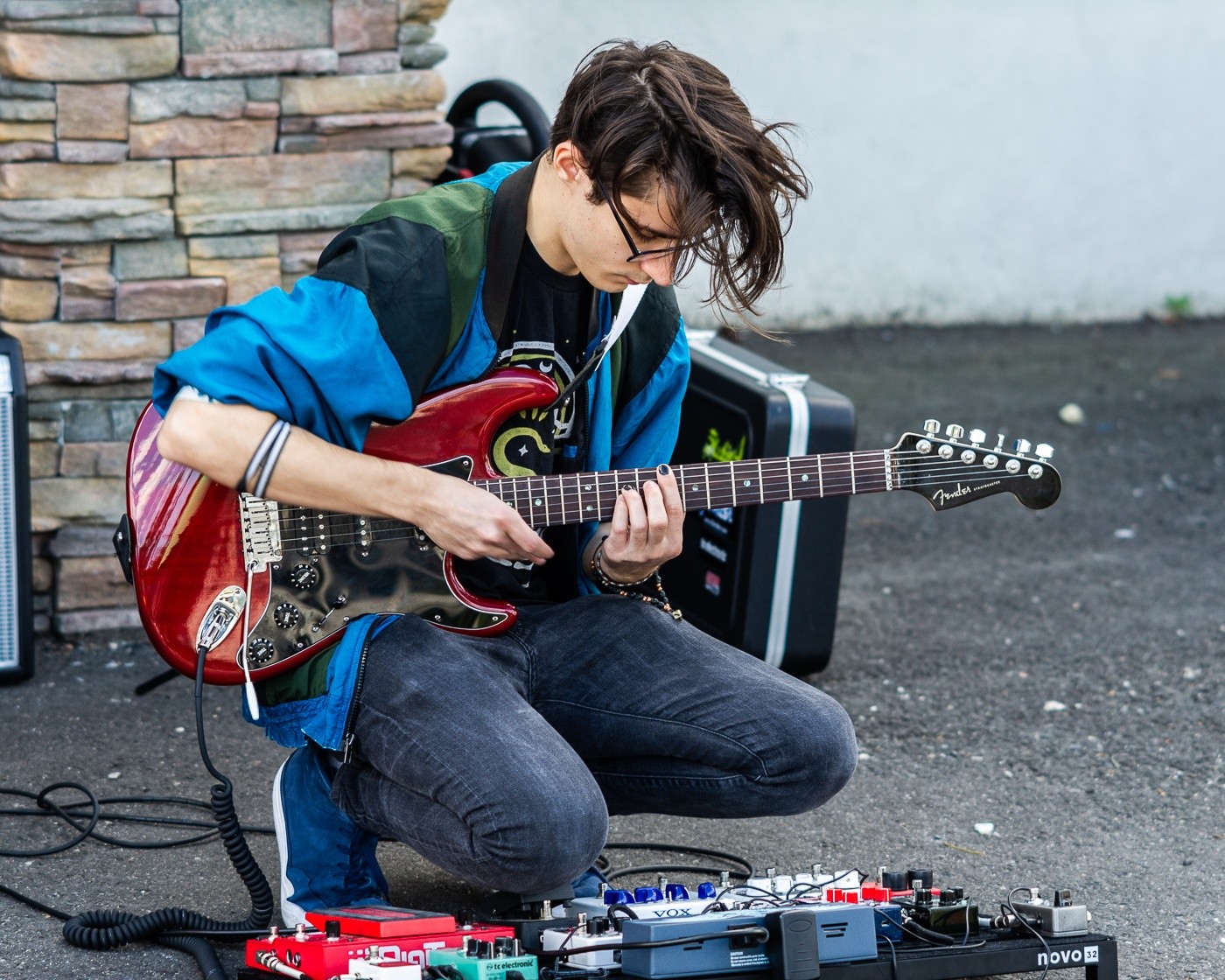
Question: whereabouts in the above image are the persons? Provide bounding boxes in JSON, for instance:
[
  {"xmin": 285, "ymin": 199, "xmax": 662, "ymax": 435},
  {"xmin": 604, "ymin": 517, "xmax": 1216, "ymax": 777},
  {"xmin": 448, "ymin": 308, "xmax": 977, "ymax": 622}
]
[{"xmin": 147, "ymin": 33, "xmax": 859, "ymax": 934}]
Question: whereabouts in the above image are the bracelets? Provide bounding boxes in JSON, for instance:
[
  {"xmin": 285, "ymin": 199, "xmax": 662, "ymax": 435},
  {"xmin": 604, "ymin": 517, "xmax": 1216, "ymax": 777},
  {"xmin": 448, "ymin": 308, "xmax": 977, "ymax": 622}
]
[{"xmin": 590, "ymin": 535, "xmax": 683, "ymax": 622}]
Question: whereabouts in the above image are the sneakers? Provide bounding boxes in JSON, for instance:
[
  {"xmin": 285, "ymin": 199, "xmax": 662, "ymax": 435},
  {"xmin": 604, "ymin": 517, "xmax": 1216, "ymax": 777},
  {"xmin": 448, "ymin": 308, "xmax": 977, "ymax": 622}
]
[
  {"xmin": 565, "ymin": 865, "xmax": 612, "ymax": 905},
  {"xmin": 273, "ymin": 746, "xmax": 394, "ymax": 933}
]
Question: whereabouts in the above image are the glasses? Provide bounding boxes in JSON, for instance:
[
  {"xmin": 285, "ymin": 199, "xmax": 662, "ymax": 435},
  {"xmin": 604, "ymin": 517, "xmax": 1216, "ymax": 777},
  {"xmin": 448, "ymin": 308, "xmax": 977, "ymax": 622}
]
[{"xmin": 578, "ymin": 142, "xmax": 735, "ymax": 262}]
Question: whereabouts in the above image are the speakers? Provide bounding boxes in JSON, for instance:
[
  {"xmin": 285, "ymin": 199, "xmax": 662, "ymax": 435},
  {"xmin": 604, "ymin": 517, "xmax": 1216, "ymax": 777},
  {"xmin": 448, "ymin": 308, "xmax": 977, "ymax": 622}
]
[{"xmin": 0, "ymin": 328, "xmax": 33, "ymax": 682}]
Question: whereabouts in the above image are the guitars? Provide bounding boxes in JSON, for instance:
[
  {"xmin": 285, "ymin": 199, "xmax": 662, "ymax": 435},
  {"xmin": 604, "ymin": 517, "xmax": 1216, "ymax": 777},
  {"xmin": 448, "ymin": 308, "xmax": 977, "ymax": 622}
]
[{"xmin": 112, "ymin": 366, "xmax": 1063, "ymax": 721}]
